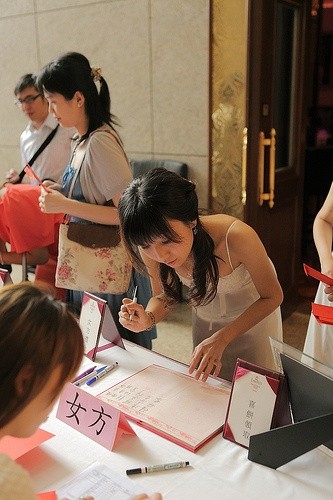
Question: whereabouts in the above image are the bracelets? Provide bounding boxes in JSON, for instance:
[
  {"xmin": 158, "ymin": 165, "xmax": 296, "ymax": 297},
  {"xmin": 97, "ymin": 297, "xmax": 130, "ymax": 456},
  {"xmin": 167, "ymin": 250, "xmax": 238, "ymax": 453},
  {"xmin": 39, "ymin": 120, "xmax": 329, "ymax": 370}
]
[{"xmin": 145, "ymin": 311, "xmax": 156, "ymax": 331}]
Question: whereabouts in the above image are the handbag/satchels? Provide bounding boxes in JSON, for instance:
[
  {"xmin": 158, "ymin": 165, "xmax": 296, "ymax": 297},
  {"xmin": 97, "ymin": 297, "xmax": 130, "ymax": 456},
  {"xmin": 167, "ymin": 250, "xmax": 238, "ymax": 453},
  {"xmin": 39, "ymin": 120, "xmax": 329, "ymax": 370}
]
[{"xmin": 55, "ymin": 222, "xmax": 135, "ymax": 296}]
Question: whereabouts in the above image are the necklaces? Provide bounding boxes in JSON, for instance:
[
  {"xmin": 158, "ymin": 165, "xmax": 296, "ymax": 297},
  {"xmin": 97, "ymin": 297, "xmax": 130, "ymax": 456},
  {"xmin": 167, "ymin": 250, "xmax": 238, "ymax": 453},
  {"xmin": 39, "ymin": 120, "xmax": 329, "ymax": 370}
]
[{"xmin": 184, "ymin": 261, "xmax": 193, "ymax": 278}]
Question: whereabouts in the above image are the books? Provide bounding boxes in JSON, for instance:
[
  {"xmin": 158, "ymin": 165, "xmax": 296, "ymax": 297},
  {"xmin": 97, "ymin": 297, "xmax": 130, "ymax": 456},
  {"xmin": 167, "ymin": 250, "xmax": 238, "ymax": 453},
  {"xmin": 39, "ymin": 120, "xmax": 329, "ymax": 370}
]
[{"xmin": 95, "ymin": 363, "xmax": 234, "ymax": 453}]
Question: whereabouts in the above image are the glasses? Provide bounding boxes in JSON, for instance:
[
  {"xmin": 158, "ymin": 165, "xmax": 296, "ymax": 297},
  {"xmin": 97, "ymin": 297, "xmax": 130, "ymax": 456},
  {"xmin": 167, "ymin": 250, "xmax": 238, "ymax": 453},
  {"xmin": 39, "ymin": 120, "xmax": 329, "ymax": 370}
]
[{"xmin": 14, "ymin": 94, "xmax": 42, "ymax": 107}]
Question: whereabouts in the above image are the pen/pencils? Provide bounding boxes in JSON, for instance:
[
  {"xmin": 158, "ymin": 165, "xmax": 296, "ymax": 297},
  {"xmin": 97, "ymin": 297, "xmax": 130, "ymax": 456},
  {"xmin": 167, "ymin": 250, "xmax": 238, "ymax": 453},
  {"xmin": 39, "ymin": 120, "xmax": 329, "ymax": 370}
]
[
  {"xmin": 70, "ymin": 362, "xmax": 118, "ymax": 389},
  {"xmin": 129, "ymin": 285, "xmax": 138, "ymax": 320},
  {"xmin": 126, "ymin": 461, "xmax": 190, "ymax": 476}
]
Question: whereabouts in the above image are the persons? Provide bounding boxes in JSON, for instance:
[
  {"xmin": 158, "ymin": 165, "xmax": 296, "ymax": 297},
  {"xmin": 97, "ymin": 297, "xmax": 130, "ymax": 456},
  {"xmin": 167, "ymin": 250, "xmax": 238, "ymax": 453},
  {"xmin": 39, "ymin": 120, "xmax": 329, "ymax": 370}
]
[
  {"xmin": 0, "ymin": 281, "xmax": 162, "ymax": 500},
  {"xmin": 301, "ymin": 181, "xmax": 333, "ymax": 369},
  {"xmin": 118, "ymin": 167, "xmax": 284, "ymax": 382},
  {"xmin": 0, "ymin": 182, "xmax": 67, "ymax": 305},
  {"xmin": 0, "ymin": 73, "xmax": 79, "ymax": 186},
  {"xmin": 32, "ymin": 52, "xmax": 157, "ymax": 351}
]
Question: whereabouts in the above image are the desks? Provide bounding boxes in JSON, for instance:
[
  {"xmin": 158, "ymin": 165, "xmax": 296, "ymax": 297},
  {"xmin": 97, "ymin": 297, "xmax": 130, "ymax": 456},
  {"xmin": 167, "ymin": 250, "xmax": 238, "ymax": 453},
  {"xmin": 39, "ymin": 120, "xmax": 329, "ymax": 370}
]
[{"xmin": 16, "ymin": 338, "xmax": 333, "ymax": 500}]
